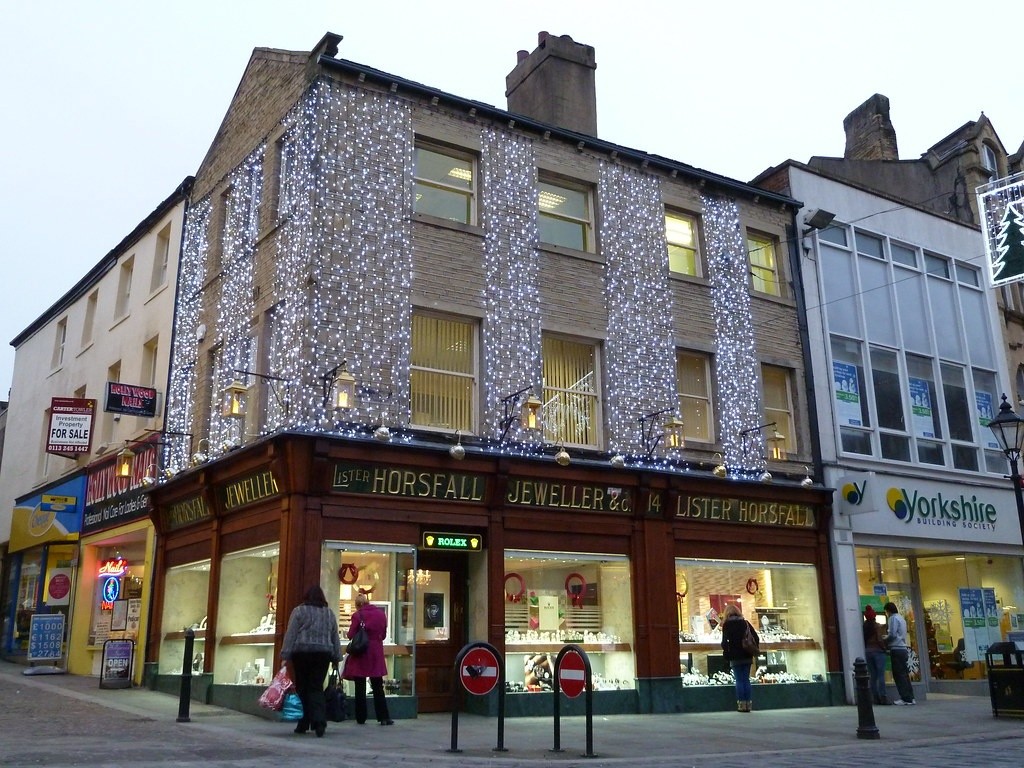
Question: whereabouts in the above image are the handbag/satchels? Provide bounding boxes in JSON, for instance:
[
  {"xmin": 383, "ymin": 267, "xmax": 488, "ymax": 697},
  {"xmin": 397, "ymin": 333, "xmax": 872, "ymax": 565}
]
[
  {"xmin": 346, "ymin": 609, "xmax": 369, "ymax": 657},
  {"xmin": 742, "ymin": 620, "xmax": 761, "ymax": 658},
  {"xmin": 259, "ymin": 666, "xmax": 293, "ymax": 711},
  {"xmin": 283, "ymin": 669, "xmax": 304, "ymax": 722},
  {"xmin": 325, "ymin": 668, "xmax": 348, "ymax": 722}
]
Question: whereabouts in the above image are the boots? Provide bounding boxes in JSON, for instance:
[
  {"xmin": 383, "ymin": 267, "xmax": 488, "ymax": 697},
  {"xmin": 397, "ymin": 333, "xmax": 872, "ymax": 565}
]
[{"xmin": 738, "ymin": 701, "xmax": 752, "ymax": 712}]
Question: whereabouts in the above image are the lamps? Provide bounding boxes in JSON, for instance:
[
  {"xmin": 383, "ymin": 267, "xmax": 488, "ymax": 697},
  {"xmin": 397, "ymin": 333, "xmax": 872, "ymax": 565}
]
[
  {"xmin": 140, "ymin": 463, "xmax": 173, "ymax": 488},
  {"xmin": 638, "ymin": 405, "xmax": 685, "ymax": 452},
  {"xmin": 192, "ymin": 438, "xmax": 221, "ymax": 465},
  {"xmin": 740, "ymin": 421, "xmax": 784, "ymax": 458},
  {"xmin": 791, "ymin": 464, "xmax": 813, "ymax": 488},
  {"xmin": 758, "ymin": 459, "xmax": 772, "ymax": 483},
  {"xmin": 220, "ymin": 369, "xmax": 291, "ymax": 418},
  {"xmin": 449, "ymin": 428, "xmax": 464, "ymax": 459},
  {"xmin": 372, "ymin": 411, "xmax": 391, "ymax": 441},
  {"xmin": 500, "ymin": 385, "xmax": 542, "ymax": 434},
  {"xmin": 610, "ymin": 441, "xmax": 624, "ymax": 468},
  {"xmin": 318, "ymin": 360, "xmax": 357, "ymax": 411},
  {"xmin": 709, "ymin": 452, "xmax": 727, "ymax": 478},
  {"xmin": 220, "ymin": 423, "xmax": 260, "ymax": 451},
  {"xmin": 95, "ymin": 440, "xmax": 122, "ymax": 454},
  {"xmin": 802, "ymin": 207, "xmax": 836, "ymax": 234},
  {"xmin": 112, "ymin": 437, "xmax": 172, "ymax": 479},
  {"xmin": 553, "ymin": 436, "xmax": 570, "ymax": 466}
]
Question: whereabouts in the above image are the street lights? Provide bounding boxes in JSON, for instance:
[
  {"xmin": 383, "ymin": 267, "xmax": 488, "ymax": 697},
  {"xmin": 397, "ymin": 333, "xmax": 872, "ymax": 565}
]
[{"xmin": 987, "ymin": 392, "xmax": 1024, "ymax": 548}]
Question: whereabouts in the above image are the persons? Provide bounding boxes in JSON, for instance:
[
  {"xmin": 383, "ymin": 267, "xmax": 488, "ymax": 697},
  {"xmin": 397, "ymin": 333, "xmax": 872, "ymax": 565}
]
[
  {"xmin": 863, "ymin": 605, "xmax": 893, "ymax": 704},
  {"xmin": 720, "ymin": 604, "xmax": 760, "ymax": 713},
  {"xmin": 280, "ymin": 585, "xmax": 343, "ymax": 738},
  {"xmin": 881, "ymin": 601, "xmax": 917, "ymax": 705},
  {"xmin": 342, "ymin": 593, "xmax": 394, "ymax": 726}
]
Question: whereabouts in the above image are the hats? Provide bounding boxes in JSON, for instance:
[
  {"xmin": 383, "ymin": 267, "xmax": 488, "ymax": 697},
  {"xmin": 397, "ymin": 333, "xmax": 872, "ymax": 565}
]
[{"xmin": 864, "ymin": 605, "xmax": 876, "ymax": 620}]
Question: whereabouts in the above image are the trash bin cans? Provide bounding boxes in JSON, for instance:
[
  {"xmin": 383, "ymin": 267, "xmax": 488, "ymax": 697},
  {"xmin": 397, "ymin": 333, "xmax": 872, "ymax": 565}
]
[{"xmin": 985, "ymin": 642, "xmax": 1024, "ymax": 717}]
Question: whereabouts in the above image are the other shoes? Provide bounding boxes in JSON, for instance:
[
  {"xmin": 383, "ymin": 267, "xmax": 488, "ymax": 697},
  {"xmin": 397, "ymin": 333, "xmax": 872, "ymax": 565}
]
[
  {"xmin": 356, "ymin": 719, "xmax": 366, "ymax": 724},
  {"xmin": 294, "ymin": 727, "xmax": 306, "ymax": 733},
  {"xmin": 876, "ymin": 701, "xmax": 894, "ymax": 705},
  {"xmin": 380, "ymin": 720, "xmax": 394, "ymax": 725},
  {"xmin": 315, "ymin": 722, "xmax": 327, "ymax": 737}
]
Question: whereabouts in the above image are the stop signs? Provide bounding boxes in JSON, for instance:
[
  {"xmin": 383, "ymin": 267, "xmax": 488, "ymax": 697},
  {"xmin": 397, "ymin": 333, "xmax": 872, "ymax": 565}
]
[
  {"xmin": 559, "ymin": 651, "xmax": 585, "ymax": 698},
  {"xmin": 459, "ymin": 648, "xmax": 499, "ymax": 696}
]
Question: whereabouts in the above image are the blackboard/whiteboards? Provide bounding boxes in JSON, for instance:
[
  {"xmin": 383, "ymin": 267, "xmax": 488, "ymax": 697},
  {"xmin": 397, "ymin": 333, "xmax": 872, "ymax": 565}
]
[
  {"xmin": 101, "ymin": 639, "xmax": 134, "ymax": 687},
  {"xmin": 26, "ymin": 615, "xmax": 66, "ymax": 660}
]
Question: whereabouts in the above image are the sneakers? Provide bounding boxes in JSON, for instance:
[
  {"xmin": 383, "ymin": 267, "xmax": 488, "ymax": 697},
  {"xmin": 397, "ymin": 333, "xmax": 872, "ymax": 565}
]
[{"xmin": 893, "ymin": 699, "xmax": 917, "ymax": 705}]
